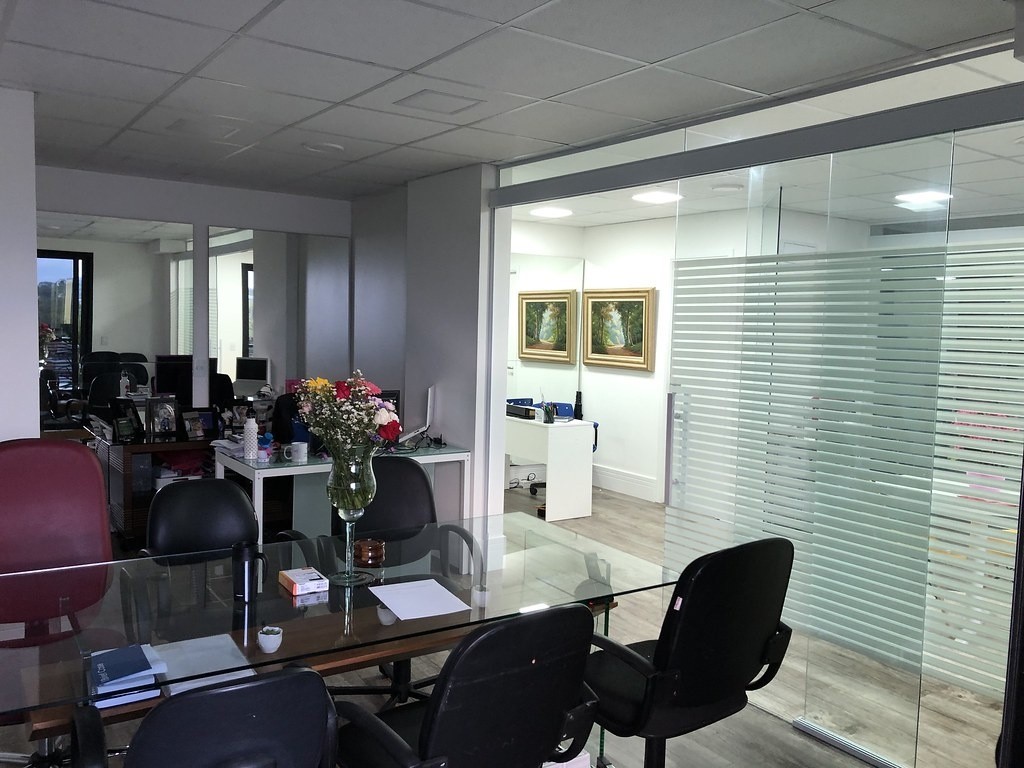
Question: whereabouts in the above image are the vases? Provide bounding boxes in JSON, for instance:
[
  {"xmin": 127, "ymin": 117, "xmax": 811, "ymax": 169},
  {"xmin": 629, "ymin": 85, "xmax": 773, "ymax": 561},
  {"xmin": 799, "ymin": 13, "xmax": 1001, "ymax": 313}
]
[{"xmin": 326, "ymin": 439, "xmax": 377, "ymax": 588}]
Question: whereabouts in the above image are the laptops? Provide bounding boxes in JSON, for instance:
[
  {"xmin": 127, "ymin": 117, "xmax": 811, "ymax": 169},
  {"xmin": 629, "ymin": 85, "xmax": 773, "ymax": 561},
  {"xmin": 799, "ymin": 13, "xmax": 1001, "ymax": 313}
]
[
  {"xmin": 231, "ymin": 357, "xmax": 270, "ymax": 397},
  {"xmin": 399, "ymin": 384, "xmax": 436, "ymax": 443}
]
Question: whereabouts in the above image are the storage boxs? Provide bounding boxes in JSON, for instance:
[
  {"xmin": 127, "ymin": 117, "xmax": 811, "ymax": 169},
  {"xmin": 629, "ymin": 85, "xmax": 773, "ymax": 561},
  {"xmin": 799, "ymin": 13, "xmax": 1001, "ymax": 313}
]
[{"xmin": 278, "ymin": 567, "xmax": 329, "ymax": 595}]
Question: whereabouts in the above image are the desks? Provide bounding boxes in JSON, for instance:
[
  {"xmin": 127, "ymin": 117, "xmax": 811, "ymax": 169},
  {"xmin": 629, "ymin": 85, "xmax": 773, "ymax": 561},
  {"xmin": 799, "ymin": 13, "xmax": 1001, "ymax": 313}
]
[
  {"xmin": 0, "ymin": 510, "xmax": 685, "ymax": 768},
  {"xmin": 211, "ymin": 443, "xmax": 473, "ymax": 593},
  {"xmin": 81, "ymin": 423, "xmax": 225, "ymax": 539},
  {"xmin": 506, "ymin": 415, "xmax": 595, "ymax": 522},
  {"xmin": 84, "ymin": 394, "xmax": 170, "ymax": 405}
]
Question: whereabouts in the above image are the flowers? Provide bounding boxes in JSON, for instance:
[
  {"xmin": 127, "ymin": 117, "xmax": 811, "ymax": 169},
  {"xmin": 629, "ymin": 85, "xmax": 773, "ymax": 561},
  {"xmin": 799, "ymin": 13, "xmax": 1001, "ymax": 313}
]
[{"xmin": 292, "ymin": 368, "xmax": 404, "ymax": 446}]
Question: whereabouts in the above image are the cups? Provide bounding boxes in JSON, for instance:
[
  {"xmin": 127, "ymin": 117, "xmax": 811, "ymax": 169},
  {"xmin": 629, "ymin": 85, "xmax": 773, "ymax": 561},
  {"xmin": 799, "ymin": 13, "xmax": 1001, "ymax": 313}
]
[{"xmin": 284, "ymin": 442, "xmax": 308, "ymax": 463}]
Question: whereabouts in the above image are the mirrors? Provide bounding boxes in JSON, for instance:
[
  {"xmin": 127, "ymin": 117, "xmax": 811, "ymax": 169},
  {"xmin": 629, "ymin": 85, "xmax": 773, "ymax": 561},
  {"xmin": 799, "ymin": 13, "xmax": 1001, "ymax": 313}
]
[{"xmin": 505, "ymin": 250, "xmax": 585, "ymax": 490}]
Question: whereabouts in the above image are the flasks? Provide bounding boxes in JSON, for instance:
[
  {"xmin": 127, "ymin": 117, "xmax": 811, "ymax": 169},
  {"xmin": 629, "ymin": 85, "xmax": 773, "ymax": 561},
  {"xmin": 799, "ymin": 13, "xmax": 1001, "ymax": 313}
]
[
  {"xmin": 243, "ymin": 407, "xmax": 259, "ymax": 460},
  {"xmin": 120, "ymin": 370, "xmax": 130, "ymax": 397}
]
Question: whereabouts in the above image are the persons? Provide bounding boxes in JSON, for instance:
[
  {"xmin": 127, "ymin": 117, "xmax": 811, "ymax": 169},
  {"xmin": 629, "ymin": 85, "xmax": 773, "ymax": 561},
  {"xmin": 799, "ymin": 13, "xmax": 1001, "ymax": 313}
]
[
  {"xmin": 233, "ymin": 407, "xmax": 246, "ymax": 425},
  {"xmin": 160, "ymin": 409, "xmax": 171, "ymax": 430}
]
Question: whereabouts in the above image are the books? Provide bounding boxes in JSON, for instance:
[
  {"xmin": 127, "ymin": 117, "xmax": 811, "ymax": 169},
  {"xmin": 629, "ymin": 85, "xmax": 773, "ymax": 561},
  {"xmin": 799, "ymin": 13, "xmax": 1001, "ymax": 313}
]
[{"xmin": 90, "ymin": 645, "xmax": 159, "ymax": 709}]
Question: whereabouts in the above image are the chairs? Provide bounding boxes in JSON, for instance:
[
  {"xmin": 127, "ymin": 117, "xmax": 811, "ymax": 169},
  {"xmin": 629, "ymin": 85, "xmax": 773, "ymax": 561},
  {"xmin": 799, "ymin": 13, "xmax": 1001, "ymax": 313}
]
[
  {"xmin": 530, "ymin": 402, "xmax": 599, "ymax": 495},
  {"xmin": 0, "ymin": 351, "xmax": 794, "ymax": 768}
]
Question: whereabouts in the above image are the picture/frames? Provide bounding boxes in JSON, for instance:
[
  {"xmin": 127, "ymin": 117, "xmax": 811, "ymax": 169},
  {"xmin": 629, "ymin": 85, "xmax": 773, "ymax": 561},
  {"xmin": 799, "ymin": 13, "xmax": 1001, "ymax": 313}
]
[
  {"xmin": 516, "ymin": 289, "xmax": 577, "ymax": 364},
  {"xmin": 580, "ymin": 286, "xmax": 655, "ymax": 372}
]
